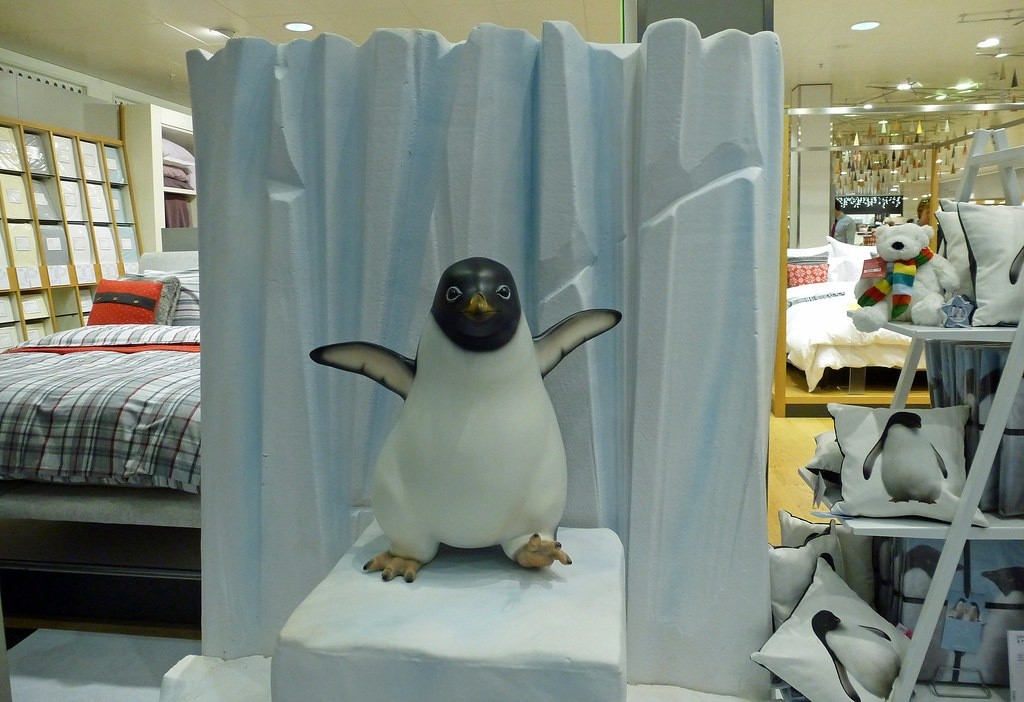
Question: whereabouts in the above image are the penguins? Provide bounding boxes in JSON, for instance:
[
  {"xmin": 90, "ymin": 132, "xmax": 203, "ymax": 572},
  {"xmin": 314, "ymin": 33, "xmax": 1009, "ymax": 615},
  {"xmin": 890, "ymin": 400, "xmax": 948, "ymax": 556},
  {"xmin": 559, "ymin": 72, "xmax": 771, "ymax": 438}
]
[
  {"xmin": 309, "ymin": 256, "xmax": 623, "ymax": 581},
  {"xmin": 978, "ymin": 566, "xmax": 1023, "ymax": 687},
  {"xmin": 811, "ymin": 610, "xmax": 901, "ymax": 702},
  {"xmin": 904, "ymin": 545, "xmax": 967, "ymax": 684},
  {"xmin": 863, "ymin": 412, "xmax": 948, "ymax": 504}
]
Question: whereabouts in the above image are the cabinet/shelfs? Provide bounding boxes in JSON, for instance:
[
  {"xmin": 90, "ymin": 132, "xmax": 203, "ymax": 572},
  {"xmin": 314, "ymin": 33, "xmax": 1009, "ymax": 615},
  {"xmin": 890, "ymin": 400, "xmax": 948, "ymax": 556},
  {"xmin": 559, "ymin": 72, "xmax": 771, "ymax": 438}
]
[{"xmin": 836, "ymin": 125, "xmax": 1024, "ymax": 702}]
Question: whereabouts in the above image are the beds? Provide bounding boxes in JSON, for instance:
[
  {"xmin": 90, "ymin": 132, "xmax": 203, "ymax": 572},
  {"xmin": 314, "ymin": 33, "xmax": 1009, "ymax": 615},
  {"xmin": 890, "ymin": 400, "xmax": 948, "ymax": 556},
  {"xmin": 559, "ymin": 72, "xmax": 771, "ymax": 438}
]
[
  {"xmin": 0, "ymin": 324, "xmax": 202, "ymax": 640},
  {"xmin": 783, "ymin": 280, "xmax": 930, "ymax": 394}
]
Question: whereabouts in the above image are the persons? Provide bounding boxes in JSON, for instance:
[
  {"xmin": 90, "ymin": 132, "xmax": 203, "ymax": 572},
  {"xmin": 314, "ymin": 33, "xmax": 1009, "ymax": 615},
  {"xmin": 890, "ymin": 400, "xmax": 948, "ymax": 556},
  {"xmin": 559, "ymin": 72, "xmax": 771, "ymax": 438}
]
[{"xmin": 832, "ymin": 199, "xmax": 858, "ymax": 245}]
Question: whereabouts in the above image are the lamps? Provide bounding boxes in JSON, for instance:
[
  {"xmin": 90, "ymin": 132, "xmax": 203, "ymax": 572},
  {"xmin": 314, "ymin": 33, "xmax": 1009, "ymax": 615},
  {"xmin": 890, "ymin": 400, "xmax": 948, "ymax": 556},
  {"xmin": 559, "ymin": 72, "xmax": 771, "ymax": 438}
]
[
  {"xmin": 828, "ymin": 57, "xmax": 1023, "ymax": 209},
  {"xmin": 211, "ymin": 29, "xmax": 234, "ymax": 40}
]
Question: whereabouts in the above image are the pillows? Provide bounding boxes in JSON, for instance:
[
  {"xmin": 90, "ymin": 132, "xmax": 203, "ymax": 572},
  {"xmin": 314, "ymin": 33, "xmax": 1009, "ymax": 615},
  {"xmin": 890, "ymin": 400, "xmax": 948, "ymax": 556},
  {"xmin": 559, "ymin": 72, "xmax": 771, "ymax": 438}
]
[
  {"xmin": 117, "ymin": 274, "xmax": 181, "ymax": 327},
  {"xmin": 934, "ymin": 211, "xmax": 976, "ymax": 306},
  {"xmin": 143, "ymin": 269, "xmax": 200, "ymax": 326},
  {"xmin": 786, "ymin": 252, "xmax": 830, "ymax": 265},
  {"xmin": 786, "ymin": 263, "xmax": 831, "ymax": 288},
  {"xmin": 765, "ymin": 519, "xmax": 846, "ymax": 632},
  {"xmin": 827, "ymin": 402, "xmax": 991, "ymax": 529},
  {"xmin": 751, "ymin": 556, "xmax": 911, "ymax": 701},
  {"xmin": 162, "ymin": 136, "xmax": 196, "ymax": 168},
  {"xmin": 829, "ymin": 236, "xmax": 878, "ymax": 281},
  {"xmin": 163, "ymin": 177, "xmax": 194, "ymax": 191},
  {"xmin": 805, "ymin": 431, "xmax": 844, "ymax": 483},
  {"xmin": 778, "ymin": 510, "xmax": 876, "ymax": 606},
  {"xmin": 87, "ymin": 279, "xmax": 163, "ymax": 327},
  {"xmin": 957, "ymin": 201, "xmax": 1024, "ymax": 327},
  {"xmin": 163, "ymin": 165, "xmax": 189, "ymax": 182},
  {"xmin": 934, "ymin": 198, "xmax": 961, "ymax": 258}
]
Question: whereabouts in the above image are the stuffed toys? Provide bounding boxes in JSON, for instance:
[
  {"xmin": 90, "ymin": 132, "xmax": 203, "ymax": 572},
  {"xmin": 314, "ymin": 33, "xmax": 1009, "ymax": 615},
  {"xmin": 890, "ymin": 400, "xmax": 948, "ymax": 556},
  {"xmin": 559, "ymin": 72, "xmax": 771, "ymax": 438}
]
[{"xmin": 852, "ymin": 223, "xmax": 960, "ymax": 332}]
